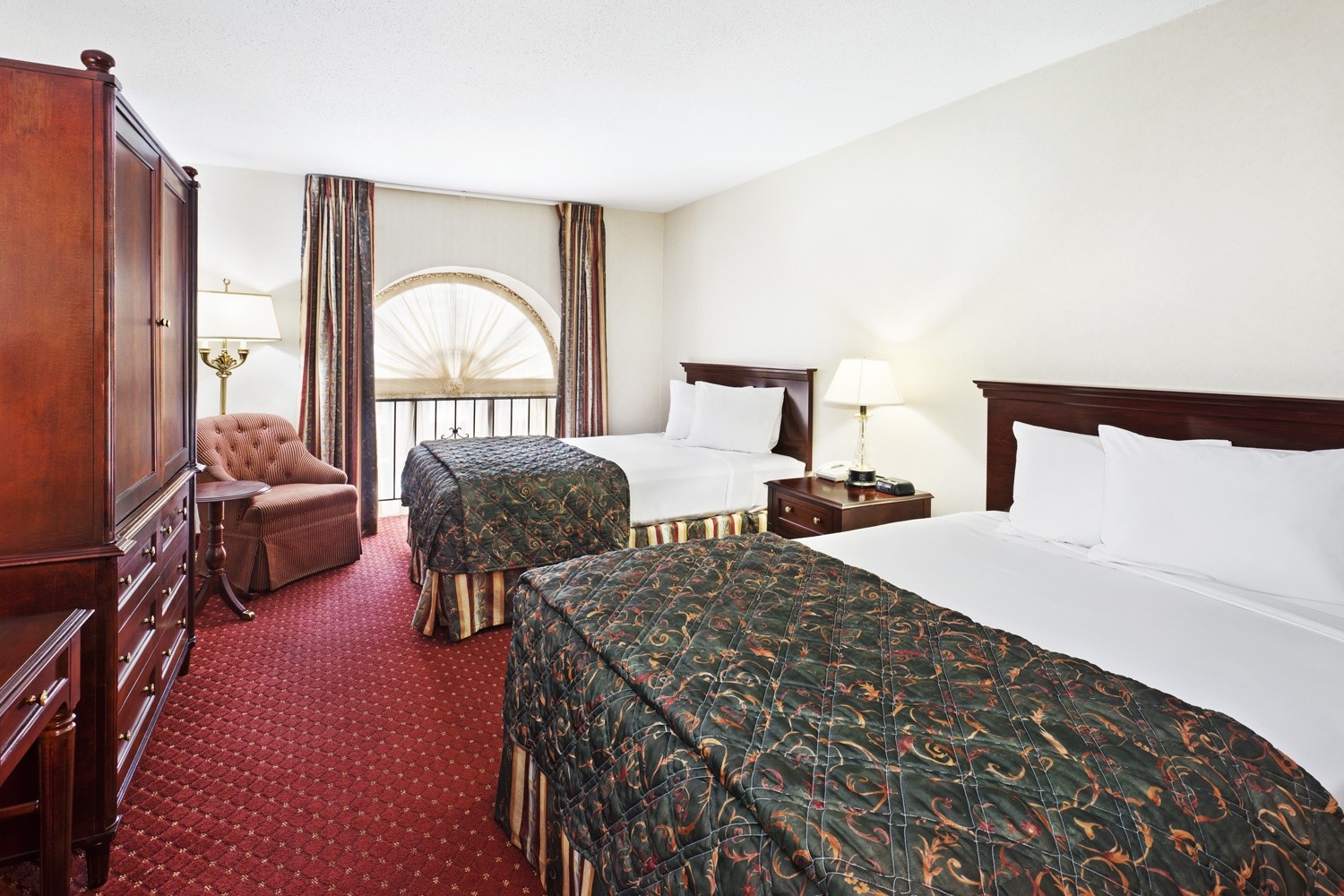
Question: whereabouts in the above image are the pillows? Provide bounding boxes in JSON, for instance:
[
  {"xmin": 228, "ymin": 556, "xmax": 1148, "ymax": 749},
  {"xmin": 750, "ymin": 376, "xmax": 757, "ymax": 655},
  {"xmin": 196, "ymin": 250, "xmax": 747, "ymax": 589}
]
[
  {"xmin": 1086, "ymin": 424, "xmax": 1344, "ymax": 608},
  {"xmin": 663, "ymin": 380, "xmax": 754, "ymax": 440},
  {"xmin": 682, "ymin": 380, "xmax": 786, "ymax": 455},
  {"xmin": 994, "ymin": 420, "xmax": 1232, "ymax": 549}
]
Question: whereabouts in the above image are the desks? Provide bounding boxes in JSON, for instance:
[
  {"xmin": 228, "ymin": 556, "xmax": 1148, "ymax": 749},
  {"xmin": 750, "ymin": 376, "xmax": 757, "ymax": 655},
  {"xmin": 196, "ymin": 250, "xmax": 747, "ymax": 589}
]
[
  {"xmin": 0, "ymin": 607, "xmax": 95, "ymax": 896},
  {"xmin": 192, "ymin": 479, "xmax": 273, "ymax": 621}
]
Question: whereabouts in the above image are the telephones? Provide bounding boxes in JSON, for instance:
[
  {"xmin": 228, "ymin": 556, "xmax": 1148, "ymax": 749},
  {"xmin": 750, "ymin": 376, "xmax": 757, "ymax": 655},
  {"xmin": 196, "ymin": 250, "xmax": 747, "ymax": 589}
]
[{"xmin": 815, "ymin": 461, "xmax": 854, "ymax": 482}]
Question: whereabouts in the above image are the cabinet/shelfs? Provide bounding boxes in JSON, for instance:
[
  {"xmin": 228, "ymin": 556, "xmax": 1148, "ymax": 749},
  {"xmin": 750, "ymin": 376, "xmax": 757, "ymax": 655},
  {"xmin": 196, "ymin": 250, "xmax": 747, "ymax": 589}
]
[{"xmin": 0, "ymin": 49, "xmax": 201, "ymax": 887}]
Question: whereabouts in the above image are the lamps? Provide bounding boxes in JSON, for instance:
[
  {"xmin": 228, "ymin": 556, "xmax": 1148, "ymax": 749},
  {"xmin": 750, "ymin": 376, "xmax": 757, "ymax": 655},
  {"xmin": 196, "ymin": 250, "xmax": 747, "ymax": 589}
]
[
  {"xmin": 196, "ymin": 279, "xmax": 282, "ymax": 417},
  {"xmin": 823, "ymin": 357, "xmax": 905, "ymax": 489}
]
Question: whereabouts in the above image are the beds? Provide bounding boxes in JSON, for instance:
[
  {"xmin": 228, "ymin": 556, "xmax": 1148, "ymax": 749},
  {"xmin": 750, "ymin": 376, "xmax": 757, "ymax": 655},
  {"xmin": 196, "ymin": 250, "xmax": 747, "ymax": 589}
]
[
  {"xmin": 401, "ymin": 361, "xmax": 817, "ymax": 643},
  {"xmin": 491, "ymin": 380, "xmax": 1344, "ymax": 896}
]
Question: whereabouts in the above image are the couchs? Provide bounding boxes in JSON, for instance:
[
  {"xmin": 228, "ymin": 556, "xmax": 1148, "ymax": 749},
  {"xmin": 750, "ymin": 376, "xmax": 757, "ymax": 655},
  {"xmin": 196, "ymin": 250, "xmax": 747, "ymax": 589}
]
[{"xmin": 195, "ymin": 412, "xmax": 364, "ymax": 601}]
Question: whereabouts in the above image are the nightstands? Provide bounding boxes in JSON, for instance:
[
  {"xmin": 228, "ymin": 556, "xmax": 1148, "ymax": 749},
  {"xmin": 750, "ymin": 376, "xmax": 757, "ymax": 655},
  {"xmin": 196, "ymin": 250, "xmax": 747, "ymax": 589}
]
[{"xmin": 763, "ymin": 477, "xmax": 935, "ymax": 539}]
